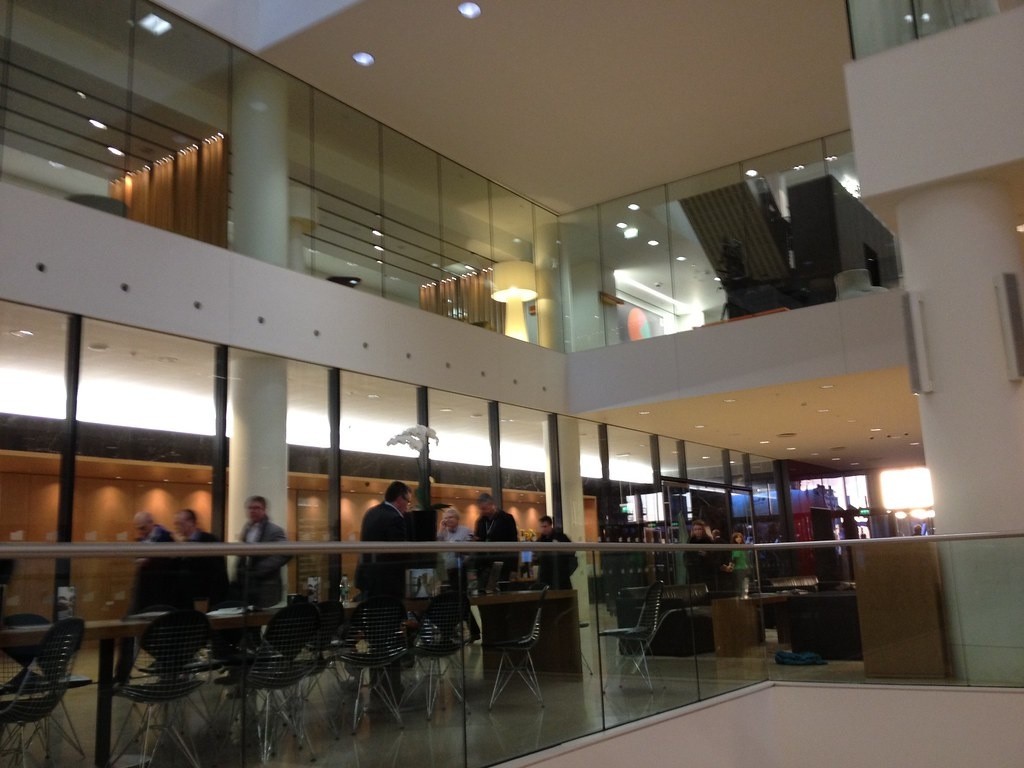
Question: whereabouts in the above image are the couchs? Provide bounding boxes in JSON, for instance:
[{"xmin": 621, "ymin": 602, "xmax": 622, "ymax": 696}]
[{"xmin": 615, "ymin": 575, "xmax": 856, "ymax": 657}]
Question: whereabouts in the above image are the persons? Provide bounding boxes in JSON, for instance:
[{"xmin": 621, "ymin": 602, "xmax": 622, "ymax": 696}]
[
  {"xmin": 712, "ymin": 530, "xmax": 732, "ymax": 580},
  {"xmin": 437, "ymin": 509, "xmax": 480, "ymax": 644},
  {"xmin": 354, "ymin": 481, "xmax": 412, "ymax": 697},
  {"xmin": 532, "ymin": 516, "xmax": 578, "ymax": 590},
  {"xmin": 682, "ymin": 520, "xmax": 715, "ymax": 586},
  {"xmin": 174, "ymin": 510, "xmax": 228, "ymax": 606},
  {"xmin": 861, "ymin": 532, "xmax": 866, "ymax": 539},
  {"xmin": 911, "ymin": 525, "xmax": 934, "ymax": 536},
  {"xmin": 472, "ymin": 493, "xmax": 518, "ymax": 592},
  {"xmin": 520, "ymin": 540, "xmax": 532, "ymax": 579},
  {"xmin": 234, "ymin": 495, "xmax": 290, "ymax": 609},
  {"xmin": 731, "ymin": 533, "xmax": 747, "ymax": 571},
  {"xmin": 113, "ymin": 512, "xmax": 177, "ymax": 684}
]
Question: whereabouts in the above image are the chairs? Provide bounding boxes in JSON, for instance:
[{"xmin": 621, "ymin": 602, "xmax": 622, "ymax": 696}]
[
  {"xmin": 2, "ymin": 592, "xmax": 470, "ymax": 767},
  {"xmin": 480, "ymin": 585, "xmax": 550, "ymax": 711}
]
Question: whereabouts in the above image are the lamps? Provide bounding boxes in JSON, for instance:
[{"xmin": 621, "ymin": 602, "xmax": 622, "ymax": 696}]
[{"xmin": 491, "ymin": 261, "xmax": 538, "ymax": 342}]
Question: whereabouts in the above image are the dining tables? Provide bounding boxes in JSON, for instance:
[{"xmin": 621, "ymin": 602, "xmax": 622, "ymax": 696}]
[{"xmin": 599, "ymin": 580, "xmax": 664, "ymax": 694}]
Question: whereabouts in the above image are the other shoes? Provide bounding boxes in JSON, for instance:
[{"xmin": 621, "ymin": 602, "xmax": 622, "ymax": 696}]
[
  {"xmin": 213, "ymin": 671, "xmax": 235, "ymax": 685},
  {"xmin": 226, "ymin": 687, "xmax": 247, "ymax": 698},
  {"xmin": 469, "ymin": 634, "xmax": 481, "ymax": 643}
]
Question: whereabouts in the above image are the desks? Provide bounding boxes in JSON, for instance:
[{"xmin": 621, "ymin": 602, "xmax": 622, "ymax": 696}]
[{"xmin": 1, "ymin": 589, "xmax": 582, "ymax": 768}]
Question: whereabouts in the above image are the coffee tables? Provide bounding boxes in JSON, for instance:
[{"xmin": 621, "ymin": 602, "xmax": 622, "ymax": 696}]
[{"xmin": 712, "ymin": 593, "xmax": 792, "ymax": 659}]
